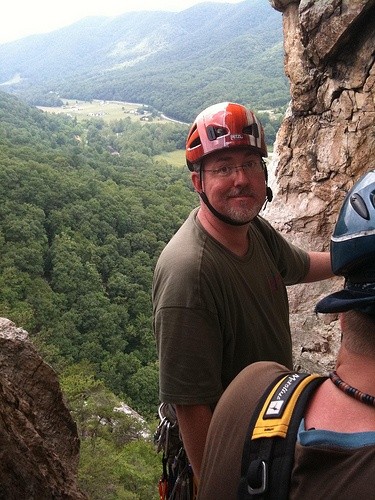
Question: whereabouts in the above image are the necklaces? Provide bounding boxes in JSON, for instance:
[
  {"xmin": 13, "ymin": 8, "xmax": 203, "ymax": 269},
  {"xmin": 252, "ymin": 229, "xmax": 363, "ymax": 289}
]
[{"xmin": 329, "ymin": 371, "xmax": 375, "ymax": 406}]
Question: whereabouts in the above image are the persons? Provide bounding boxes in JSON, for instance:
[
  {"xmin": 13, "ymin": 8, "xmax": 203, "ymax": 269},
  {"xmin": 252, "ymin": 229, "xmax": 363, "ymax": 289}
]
[
  {"xmin": 197, "ymin": 168, "xmax": 375, "ymax": 500},
  {"xmin": 152, "ymin": 101, "xmax": 337, "ymax": 500}
]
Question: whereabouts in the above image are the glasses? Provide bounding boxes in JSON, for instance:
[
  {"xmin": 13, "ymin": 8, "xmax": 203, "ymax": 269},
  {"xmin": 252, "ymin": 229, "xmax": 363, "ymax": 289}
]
[{"xmin": 201, "ymin": 160, "xmax": 266, "ymax": 176}]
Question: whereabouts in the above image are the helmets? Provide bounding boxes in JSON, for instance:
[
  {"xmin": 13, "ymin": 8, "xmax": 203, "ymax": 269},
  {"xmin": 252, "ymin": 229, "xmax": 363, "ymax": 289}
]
[
  {"xmin": 314, "ymin": 169, "xmax": 375, "ymax": 313},
  {"xmin": 185, "ymin": 102, "xmax": 267, "ymax": 172}
]
